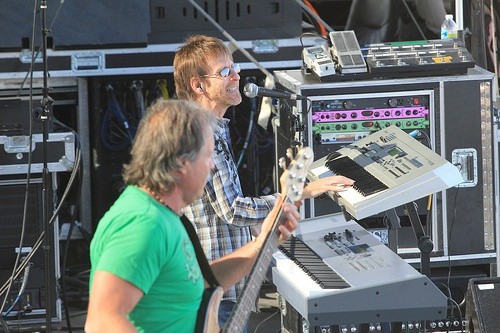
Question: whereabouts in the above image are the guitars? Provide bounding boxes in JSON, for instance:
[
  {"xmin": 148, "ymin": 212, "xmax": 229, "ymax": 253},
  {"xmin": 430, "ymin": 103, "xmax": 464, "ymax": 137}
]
[{"xmin": 195, "ymin": 143, "xmax": 315, "ymax": 333}]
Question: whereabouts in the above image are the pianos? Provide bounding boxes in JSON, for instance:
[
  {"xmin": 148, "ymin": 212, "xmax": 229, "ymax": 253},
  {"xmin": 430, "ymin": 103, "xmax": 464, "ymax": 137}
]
[
  {"xmin": 264, "ymin": 208, "xmax": 447, "ymax": 333},
  {"xmin": 307, "ymin": 124, "xmax": 465, "ymax": 275}
]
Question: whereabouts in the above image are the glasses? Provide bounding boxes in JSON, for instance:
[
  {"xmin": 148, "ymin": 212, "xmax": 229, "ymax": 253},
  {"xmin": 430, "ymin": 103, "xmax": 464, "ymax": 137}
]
[{"xmin": 198, "ymin": 64, "xmax": 241, "ymax": 80}]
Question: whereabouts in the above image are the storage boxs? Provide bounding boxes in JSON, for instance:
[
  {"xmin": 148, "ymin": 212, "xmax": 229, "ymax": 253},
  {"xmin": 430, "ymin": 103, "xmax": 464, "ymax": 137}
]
[{"xmin": 0, "ymin": 121, "xmax": 75, "ymax": 324}]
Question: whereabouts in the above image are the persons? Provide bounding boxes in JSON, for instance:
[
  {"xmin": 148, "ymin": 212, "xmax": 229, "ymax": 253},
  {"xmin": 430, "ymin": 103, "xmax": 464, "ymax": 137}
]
[
  {"xmin": 84, "ymin": 99, "xmax": 301, "ymax": 333},
  {"xmin": 174, "ymin": 34, "xmax": 356, "ymax": 333}
]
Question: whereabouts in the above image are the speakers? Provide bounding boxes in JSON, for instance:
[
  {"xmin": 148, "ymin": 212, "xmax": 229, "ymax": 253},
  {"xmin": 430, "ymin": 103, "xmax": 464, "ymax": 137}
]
[
  {"xmin": 0, "ymin": 174, "xmax": 63, "ymax": 324},
  {"xmin": 467, "ymin": 276, "xmax": 500, "ymax": 333}
]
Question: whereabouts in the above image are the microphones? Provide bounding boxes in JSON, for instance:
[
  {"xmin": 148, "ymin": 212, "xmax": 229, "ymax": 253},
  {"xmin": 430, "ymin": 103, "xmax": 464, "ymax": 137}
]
[{"xmin": 243, "ymin": 83, "xmax": 306, "ymax": 101}]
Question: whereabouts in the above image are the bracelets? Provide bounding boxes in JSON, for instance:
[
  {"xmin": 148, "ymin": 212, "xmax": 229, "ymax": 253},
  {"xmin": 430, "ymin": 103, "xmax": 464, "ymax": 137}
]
[{"xmin": 250, "ymin": 241, "xmax": 260, "ymax": 253}]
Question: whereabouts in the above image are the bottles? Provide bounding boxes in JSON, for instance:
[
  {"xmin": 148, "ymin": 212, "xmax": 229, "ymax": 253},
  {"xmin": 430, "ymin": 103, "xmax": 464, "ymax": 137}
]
[{"xmin": 440, "ymin": 14, "xmax": 458, "ymax": 41}]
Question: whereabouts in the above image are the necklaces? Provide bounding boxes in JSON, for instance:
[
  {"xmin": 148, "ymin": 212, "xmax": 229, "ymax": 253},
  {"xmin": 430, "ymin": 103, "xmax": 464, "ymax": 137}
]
[{"xmin": 144, "ymin": 187, "xmax": 169, "ymax": 208}]
